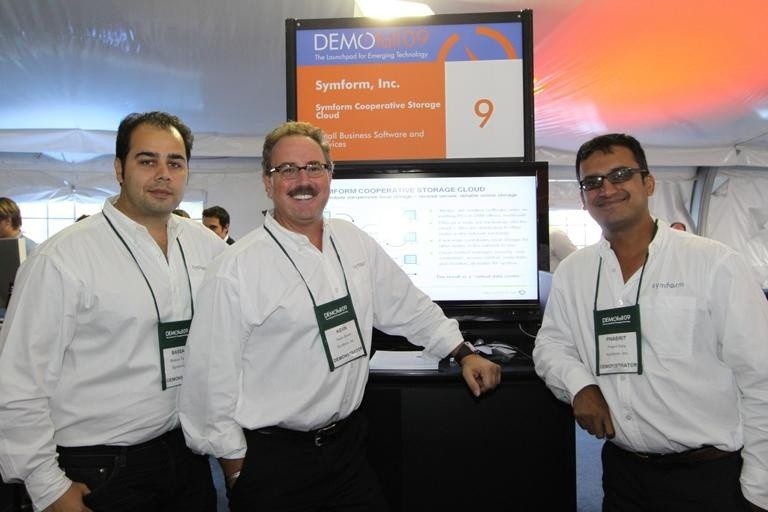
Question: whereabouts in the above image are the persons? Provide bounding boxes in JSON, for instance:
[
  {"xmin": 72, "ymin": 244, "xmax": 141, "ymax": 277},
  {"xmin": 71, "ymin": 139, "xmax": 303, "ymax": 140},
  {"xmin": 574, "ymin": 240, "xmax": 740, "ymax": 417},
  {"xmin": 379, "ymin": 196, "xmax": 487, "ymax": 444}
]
[
  {"xmin": 177, "ymin": 120, "xmax": 502, "ymax": 512},
  {"xmin": 202, "ymin": 206, "xmax": 236, "ymax": 245},
  {"xmin": 1, "ymin": 197, "xmax": 36, "ymax": 319},
  {"xmin": 670, "ymin": 222, "xmax": 685, "ymax": 231},
  {"xmin": 0, "ymin": 111, "xmax": 232, "ymax": 512},
  {"xmin": 172, "ymin": 210, "xmax": 188, "ymax": 217},
  {"xmin": 532, "ymin": 132, "xmax": 768, "ymax": 511},
  {"xmin": 548, "ymin": 228, "xmax": 577, "ymax": 272}
]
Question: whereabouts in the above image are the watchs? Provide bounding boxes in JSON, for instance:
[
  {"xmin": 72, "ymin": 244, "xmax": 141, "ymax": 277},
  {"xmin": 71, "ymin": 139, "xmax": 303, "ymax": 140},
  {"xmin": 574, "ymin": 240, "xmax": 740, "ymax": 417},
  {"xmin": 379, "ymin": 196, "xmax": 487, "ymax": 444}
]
[{"xmin": 454, "ymin": 341, "xmax": 475, "ymax": 363}]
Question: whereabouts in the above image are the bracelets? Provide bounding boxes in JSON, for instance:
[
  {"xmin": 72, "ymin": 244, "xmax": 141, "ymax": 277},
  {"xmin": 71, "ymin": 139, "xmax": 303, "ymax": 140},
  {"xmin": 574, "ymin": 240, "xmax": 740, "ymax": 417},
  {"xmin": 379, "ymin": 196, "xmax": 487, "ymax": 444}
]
[{"xmin": 225, "ymin": 471, "xmax": 240, "ymax": 479}]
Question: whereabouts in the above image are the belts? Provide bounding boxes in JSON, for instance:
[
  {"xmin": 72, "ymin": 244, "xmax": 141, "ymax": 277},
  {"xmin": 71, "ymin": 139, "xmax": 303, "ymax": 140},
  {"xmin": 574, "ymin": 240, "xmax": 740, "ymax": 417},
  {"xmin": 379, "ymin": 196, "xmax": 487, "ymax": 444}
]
[
  {"xmin": 258, "ymin": 419, "xmax": 348, "ymax": 447},
  {"xmin": 634, "ymin": 446, "xmax": 740, "ymax": 470},
  {"xmin": 64, "ymin": 427, "xmax": 185, "ymax": 468}
]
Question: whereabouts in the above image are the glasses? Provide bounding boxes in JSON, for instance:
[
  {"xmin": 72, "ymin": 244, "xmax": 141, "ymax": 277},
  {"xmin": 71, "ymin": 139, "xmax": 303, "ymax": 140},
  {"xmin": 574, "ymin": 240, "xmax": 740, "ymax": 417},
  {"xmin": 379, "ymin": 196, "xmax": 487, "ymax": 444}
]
[
  {"xmin": 579, "ymin": 167, "xmax": 647, "ymax": 191},
  {"xmin": 270, "ymin": 162, "xmax": 329, "ymax": 180}
]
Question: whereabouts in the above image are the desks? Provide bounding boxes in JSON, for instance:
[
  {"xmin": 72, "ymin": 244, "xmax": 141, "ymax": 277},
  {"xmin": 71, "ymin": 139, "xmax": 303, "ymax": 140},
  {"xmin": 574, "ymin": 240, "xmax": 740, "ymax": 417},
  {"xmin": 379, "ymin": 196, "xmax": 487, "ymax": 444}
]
[{"xmin": 355, "ymin": 339, "xmax": 576, "ymax": 509}]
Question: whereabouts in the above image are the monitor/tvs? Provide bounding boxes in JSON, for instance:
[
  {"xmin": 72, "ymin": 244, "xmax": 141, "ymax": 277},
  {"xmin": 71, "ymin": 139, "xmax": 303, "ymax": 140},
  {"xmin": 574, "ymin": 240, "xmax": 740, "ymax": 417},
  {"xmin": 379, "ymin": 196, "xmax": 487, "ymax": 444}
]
[{"xmin": 316, "ymin": 160, "xmax": 550, "ymax": 322}]
